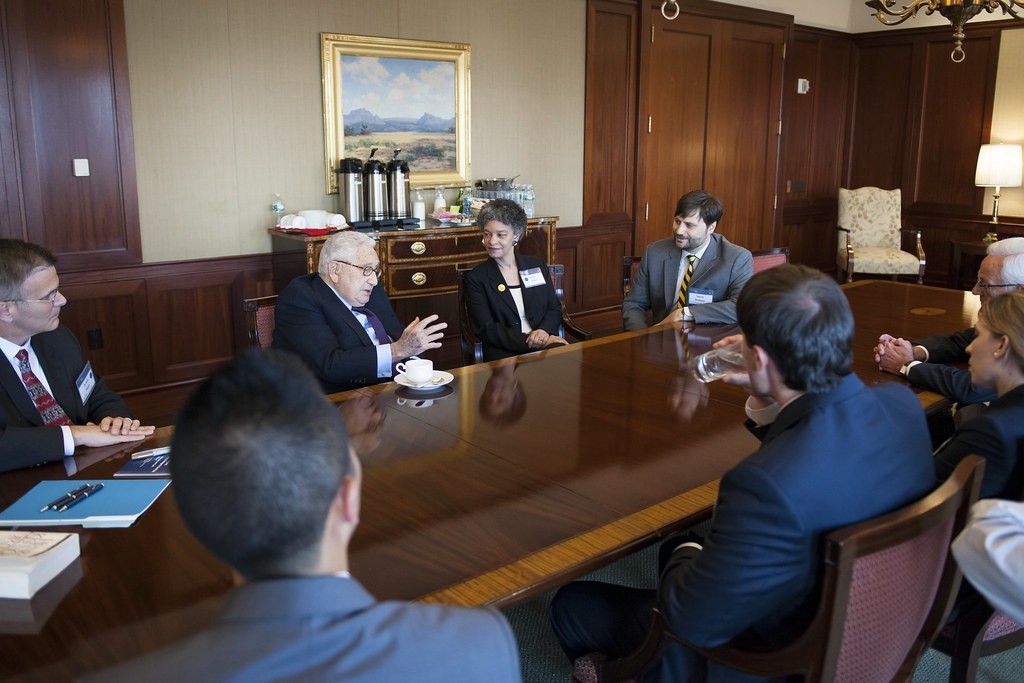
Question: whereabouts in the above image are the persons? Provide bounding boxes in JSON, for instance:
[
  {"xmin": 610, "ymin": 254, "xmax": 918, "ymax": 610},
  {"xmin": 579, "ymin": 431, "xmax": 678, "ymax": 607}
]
[
  {"xmin": 479, "ymin": 356, "xmax": 526, "ymax": 428},
  {"xmin": 641, "ymin": 324, "xmax": 733, "ymax": 425},
  {"xmin": 873, "ymin": 237, "xmax": 1024, "ymax": 628},
  {"xmin": 549, "ymin": 264, "xmax": 938, "ymax": 683},
  {"xmin": 91, "ymin": 347, "xmax": 523, "ymax": 683},
  {"xmin": 619, "ymin": 190, "xmax": 754, "ymax": 332},
  {"xmin": 271, "ymin": 231, "xmax": 447, "ymax": 396},
  {"xmin": 0, "ymin": 239, "xmax": 155, "ymax": 473},
  {"xmin": 463, "ymin": 198, "xmax": 570, "ymax": 366}
]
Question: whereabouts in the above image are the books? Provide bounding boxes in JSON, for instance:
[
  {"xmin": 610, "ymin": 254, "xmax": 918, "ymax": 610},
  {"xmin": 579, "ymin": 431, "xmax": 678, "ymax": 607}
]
[{"xmin": 0, "ymin": 530, "xmax": 81, "ymax": 599}]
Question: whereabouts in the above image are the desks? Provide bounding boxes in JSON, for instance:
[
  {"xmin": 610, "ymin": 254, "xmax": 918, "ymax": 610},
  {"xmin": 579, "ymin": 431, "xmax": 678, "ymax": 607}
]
[{"xmin": 0, "ymin": 283, "xmax": 978, "ymax": 683}]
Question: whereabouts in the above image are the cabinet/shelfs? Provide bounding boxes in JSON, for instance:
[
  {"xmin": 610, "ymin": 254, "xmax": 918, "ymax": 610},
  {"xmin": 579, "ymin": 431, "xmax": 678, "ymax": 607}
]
[
  {"xmin": 297, "ymin": 222, "xmax": 554, "ymax": 371},
  {"xmin": 241, "ymin": 293, "xmax": 280, "ymax": 355},
  {"xmin": 456, "ymin": 264, "xmax": 595, "ymax": 369},
  {"xmin": 775, "ymin": 20, "xmax": 1003, "ymax": 217},
  {"xmin": 900, "ymin": 216, "xmax": 1024, "ymax": 292},
  {"xmin": 580, "ymin": 0, "xmax": 777, "ymax": 274},
  {"xmin": 0, "ymin": 0, "xmax": 143, "ymax": 274}
]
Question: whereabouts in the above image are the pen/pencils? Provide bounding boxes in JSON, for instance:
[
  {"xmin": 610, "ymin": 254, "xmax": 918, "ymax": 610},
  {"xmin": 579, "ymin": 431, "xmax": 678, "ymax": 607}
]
[
  {"xmin": 41, "ymin": 483, "xmax": 90, "ymax": 512},
  {"xmin": 53, "ymin": 486, "xmax": 93, "ymax": 510},
  {"xmin": 59, "ymin": 483, "xmax": 104, "ymax": 512}
]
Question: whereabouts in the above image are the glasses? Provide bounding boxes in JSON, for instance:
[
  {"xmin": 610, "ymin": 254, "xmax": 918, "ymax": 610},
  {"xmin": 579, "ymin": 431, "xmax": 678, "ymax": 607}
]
[
  {"xmin": 979, "ymin": 277, "xmax": 1019, "ymax": 293},
  {"xmin": 332, "ymin": 259, "xmax": 382, "ymax": 277},
  {"xmin": 3, "ymin": 288, "xmax": 58, "ymax": 305}
]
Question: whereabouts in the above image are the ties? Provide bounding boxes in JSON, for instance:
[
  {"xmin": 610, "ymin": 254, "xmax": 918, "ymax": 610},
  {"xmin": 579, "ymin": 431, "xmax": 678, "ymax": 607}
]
[
  {"xmin": 353, "ymin": 307, "xmax": 403, "ymax": 377},
  {"xmin": 677, "ymin": 255, "xmax": 696, "ymax": 309},
  {"xmin": 15, "ymin": 349, "xmax": 74, "ymax": 426}
]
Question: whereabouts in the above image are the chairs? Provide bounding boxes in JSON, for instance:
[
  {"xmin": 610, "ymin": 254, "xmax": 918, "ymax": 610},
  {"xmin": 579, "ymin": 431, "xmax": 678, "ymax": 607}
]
[
  {"xmin": 572, "ymin": 457, "xmax": 988, "ymax": 683},
  {"xmin": 928, "ymin": 579, "xmax": 1024, "ymax": 683},
  {"xmin": 834, "ymin": 186, "xmax": 926, "ymax": 284}
]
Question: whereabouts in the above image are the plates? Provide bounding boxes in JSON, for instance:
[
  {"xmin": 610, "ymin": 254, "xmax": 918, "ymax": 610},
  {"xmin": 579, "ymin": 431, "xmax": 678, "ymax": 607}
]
[
  {"xmin": 393, "ymin": 387, "xmax": 454, "ymax": 400},
  {"xmin": 394, "ymin": 371, "xmax": 455, "ymax": 390}
]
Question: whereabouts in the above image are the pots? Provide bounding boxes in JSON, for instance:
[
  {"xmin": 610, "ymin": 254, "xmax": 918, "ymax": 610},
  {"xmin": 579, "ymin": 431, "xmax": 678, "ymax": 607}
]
[{"xmin": 480, "ymin": 174, "xmax": 520, "ymax": 190}]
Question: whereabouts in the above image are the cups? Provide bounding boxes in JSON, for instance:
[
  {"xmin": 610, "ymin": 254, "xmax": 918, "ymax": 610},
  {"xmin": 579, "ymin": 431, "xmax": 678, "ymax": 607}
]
[
  {"xmin": 396, "ymin": 359, "xmax": 433, "ymax": 383},
  {"xmin": 280, "ymin": 209, "xmax": 345, "ymax": 227},
  {"xmin": 690, "ymin": 340, "xmax": 746, "ymax": 383},
  {"xmin": 397, "ymin": 397, "xmax": 434, "ymax": 408}
]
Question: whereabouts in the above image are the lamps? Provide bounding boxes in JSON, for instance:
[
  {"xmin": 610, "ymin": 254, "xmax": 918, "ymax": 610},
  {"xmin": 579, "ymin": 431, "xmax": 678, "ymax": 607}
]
[
  {"xmin": 974, "ymin": 144, "xmax": 1022, "ymax": 222},
  {"xmin": 863, "ymin": 0, "xmax": 1024, "ymax": 63}
]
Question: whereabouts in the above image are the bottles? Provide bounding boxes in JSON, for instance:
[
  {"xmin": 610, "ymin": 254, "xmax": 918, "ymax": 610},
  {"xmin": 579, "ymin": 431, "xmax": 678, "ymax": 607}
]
[
  {"xmin": 476, "ymin": 184, "xmax": 535, "ymax": 218},
  {"xmin": 456, "ymin": 188, "xmax": 472, "ymax": 214},
  {"xmin": 433, "ymin": 190, "xmax": 446, "ymax": 212},
  {"xmin": 413, "ymin": 187, "xmax": 426, "ymax": 220}
]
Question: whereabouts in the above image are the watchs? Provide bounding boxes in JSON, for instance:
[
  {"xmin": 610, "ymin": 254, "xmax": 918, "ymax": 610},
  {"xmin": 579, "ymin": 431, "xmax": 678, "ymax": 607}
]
[{"xmin": 899, "ymin": 360, "xmax": 915, "ymax": 377}]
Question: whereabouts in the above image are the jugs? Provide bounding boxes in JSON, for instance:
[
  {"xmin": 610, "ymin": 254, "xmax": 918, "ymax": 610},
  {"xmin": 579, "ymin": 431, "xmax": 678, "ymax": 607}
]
[{"xmin": 335, "ymin": 158, "xmax": 410, "ymax": 224}]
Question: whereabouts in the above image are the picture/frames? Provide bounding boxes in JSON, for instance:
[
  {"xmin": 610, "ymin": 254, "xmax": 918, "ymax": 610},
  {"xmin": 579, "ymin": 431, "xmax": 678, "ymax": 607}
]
[{"xmin": 320, "ymin": 32, "xmax": 470, "ymax": 194}]
